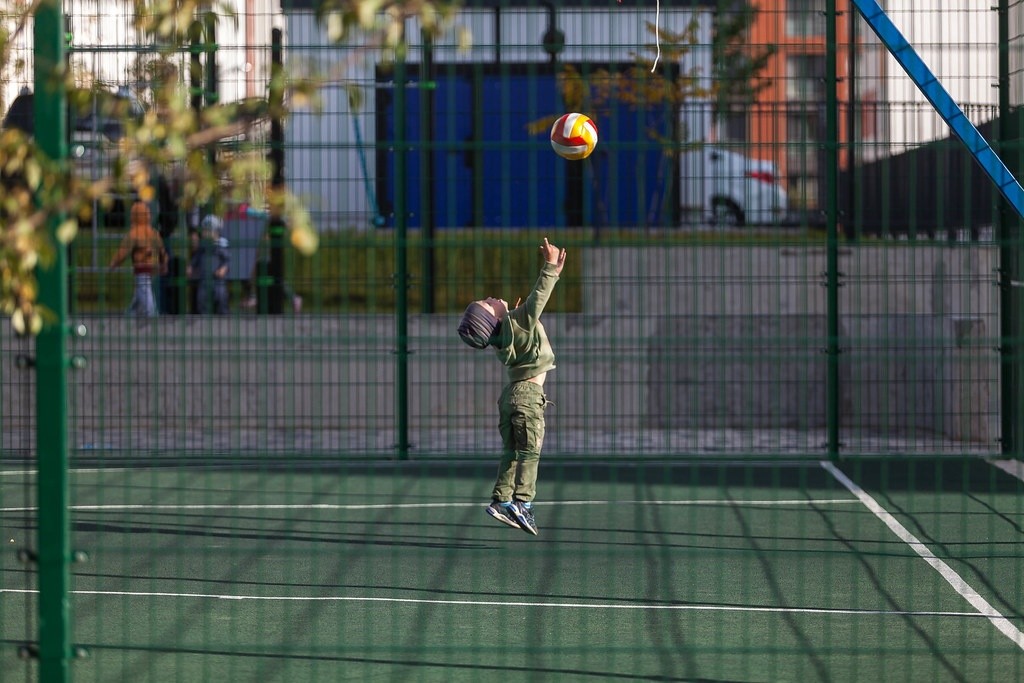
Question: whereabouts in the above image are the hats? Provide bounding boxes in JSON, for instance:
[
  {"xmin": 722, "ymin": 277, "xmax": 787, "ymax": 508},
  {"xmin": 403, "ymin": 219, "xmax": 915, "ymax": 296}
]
[
  {"xmin": 457, "ymin": 302, "xmax": 498, "ymax": 349},
  {"xmin": 201, "ymin": 214, "xmax": 225, "ymax": 230}
]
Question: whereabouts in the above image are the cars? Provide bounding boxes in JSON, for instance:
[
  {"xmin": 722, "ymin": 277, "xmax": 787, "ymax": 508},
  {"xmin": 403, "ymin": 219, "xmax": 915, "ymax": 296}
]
[{"xmin": 711, "ymin": 146, "xmax": 786, "ymax": 229}]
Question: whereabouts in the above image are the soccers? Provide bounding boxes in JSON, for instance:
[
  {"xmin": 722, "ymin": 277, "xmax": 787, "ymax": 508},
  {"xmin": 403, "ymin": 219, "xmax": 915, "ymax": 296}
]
[{"xmin": 550, "ymin": 111, "xmax": 600, "ymax": 161}]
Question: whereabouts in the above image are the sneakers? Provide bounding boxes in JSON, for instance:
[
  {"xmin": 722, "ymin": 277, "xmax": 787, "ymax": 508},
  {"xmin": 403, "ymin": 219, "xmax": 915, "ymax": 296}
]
[
  {"xmin": 507, "ymin": 501, "xmax": 538, "ymax": 538},
  {"xmin": 485, "ymin": 499, "xmax": 521, "ymax": 530}
]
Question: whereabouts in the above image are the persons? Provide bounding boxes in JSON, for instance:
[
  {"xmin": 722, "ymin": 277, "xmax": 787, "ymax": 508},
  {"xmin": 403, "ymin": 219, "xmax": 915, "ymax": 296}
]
[
  {"xmin": 458, "ymin": 237, "xmax": 567, "ymax": 536},
  {"xmin": 105, "ymin": 202, "xmax": 168, "ymax": 318},
  {"xmin": 185, "ymin": 214, "xmax": 231, "ymax": 314}
]
